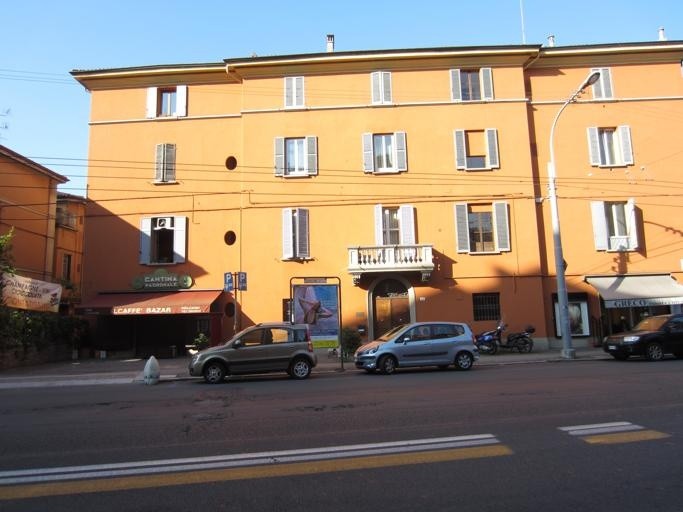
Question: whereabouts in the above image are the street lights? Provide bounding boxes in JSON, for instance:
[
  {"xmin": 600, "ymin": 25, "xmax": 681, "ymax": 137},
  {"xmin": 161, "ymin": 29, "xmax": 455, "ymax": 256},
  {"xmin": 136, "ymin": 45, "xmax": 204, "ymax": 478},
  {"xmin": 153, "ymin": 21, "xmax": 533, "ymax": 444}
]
[{"xmin": 542, "ymin": 67, "xmax": 604, "ymax": 360}]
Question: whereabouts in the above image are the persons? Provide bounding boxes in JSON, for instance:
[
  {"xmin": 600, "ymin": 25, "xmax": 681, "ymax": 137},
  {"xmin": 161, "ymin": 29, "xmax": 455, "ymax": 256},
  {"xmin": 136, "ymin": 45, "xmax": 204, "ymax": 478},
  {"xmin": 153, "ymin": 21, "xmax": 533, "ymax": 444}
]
[
  {"xmin": 617, "ymin": 316, "xmax": 630, "ymax": 331},
  {"xmin": 568, "ymin": 303, "xmax": 584, "ymax": 334},
  {"xmin": 297, "ymin": 295, "xmax": 333, "ymax": 325}
]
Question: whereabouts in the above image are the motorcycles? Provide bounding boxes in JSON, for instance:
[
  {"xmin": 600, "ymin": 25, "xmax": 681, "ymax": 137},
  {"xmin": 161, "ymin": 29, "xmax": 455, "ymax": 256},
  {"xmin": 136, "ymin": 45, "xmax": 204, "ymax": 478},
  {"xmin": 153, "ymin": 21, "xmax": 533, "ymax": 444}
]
[
  {"xmin": 471, "ymin": 332, "xmax": 498, "ymax": 356},
  {"xmin": 477, "ymin": 318, "xmax": 536, "ymax": 355}
]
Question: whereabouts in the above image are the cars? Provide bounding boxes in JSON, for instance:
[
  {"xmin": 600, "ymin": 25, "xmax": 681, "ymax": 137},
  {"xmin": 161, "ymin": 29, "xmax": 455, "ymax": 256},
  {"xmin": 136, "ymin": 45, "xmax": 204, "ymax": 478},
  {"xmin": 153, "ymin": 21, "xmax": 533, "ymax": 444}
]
[
  {"xmin": 600, "ymin": 310, "xmax": 682, "ymax": 362},
  {"xmin": 351, "ymin": 319, "xmax": 481, "ymax": 377},
  {"xmin": 186, "ymin": 320, "xmax": 320, "ymax": 386}
]
